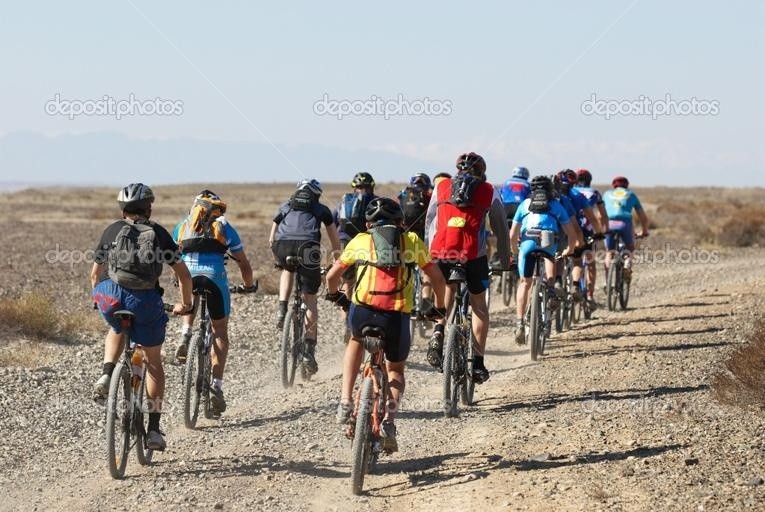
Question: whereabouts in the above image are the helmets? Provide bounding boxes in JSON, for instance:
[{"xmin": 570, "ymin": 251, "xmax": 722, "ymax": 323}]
[
  {"xmin": 196, "ymin": 190, "xmax": 221, "ymax": 202},
  {"xmin": 300, "ymin": 177, "xmax": 322, "ymax": 191},
  {"xmin": 116, "ymin": 181, "xmax": 156, "ymax": 214},
  {"xmin": 556, "ymin": 168, "xmax": 577, "ymax": 185},
  {"xmin": 351, "ymin": 171, "xmax": 376, "ymax": 189},
  {"xmin": 512, "ymin": 166, "xmax": 530, "ymax": 179},
  {"xmin": 611, "ymin": 175, "xmax": 629, "ymax": 189},
  {"xmin": 453, "ymin": 151, "xmax": 488, "ymax": 177},
  {"xmin": 555, "ymin": 177, "xmax": 570, "ymax": 195},
  {"xmin": 410, "ymin": 171, "xmax": 431, "ymax": 191},
  {"xmin": 576, "ymin": 168, "xmax": 592, "ymax": 183},
  {"xmin": 365, "ymin": 197, "xmax": 405, "ymax": 225},
  {"xmin": 531, "ymin": 176, "xmax": 553, "ymax": 191}
]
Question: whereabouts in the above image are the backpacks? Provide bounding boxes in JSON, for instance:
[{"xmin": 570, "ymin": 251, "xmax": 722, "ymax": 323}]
[
  {"xmin": 175, "ymin": 194, "xmax": 229, "ymax": 257},
  {"xmin": 529, "ymin": 189, "xmax": 552, "ymax": 213},
  {"xmin": 400, "ymin": 186, "xmax": 426, "ymax": 228},
  {"xmin": 336, "ymin": 191, "xmax": 368, "ymax": 236},
  {"xmin": 105, "ymin": 223, "xmax": 165, "ymax": 293},
  {"xmin": 365, "ymin": 224, "xmax": 406, "ymax": 270},
  {"xmin": 289, "ymin": 185, "xmax": 319, "ymax": 212},
  {"xmin": 449, "ymin": 174, "xmax": 486, "ymax": 208}
]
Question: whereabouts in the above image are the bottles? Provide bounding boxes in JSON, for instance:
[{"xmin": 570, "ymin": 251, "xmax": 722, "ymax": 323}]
[{"xmin": 131, "ymin": 343, "xmax": 143, "ymax": 387}]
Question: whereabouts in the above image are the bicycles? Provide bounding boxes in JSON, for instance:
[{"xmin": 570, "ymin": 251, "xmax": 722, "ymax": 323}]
[
  {"xmin": 325, "ymin": 291, "xmax": 448, "ymax": 497},
  {"xmin": 173, "ymin": 277, "xmax": 259, "ymax": 430},
  {"xmin": 93, "ymin": 301, "xmax": 196, "ymax": 479},
  {"xmin": 271, "ymin": 261, "xmax": 332, "ymax": 391}
]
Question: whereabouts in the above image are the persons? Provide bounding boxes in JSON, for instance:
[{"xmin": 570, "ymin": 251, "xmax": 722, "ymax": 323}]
[
  {"xmin": 172, "ymin": 190, "xmax": 254, "ymax": 413},
  {"xmin": 397, "ymin": 173, "xmax": 433, "ymax": 321},
  {"xmin": 492, "ymin": 167, "xmax": 531, "ymax": 276},
  {"xmin": 563, "ymin": 169, "xmax": 602, "ymax": 299},
  {"xmin": 325, "ymin": 197, "xmax": 446, "ymax": 454},
  {"xmin": 424, "ymin": 152, "xmax": 511, "ymax": 384},
  {"xmin": 573, "ymin": 169, "xmax": 610, "ymax": 311},
  {"xmin": 269, "ymin": 179, "xmax": 341, "ymax": 376},
  {"xmin": 90, "ymin": 182, "xmax": 194, "ymax": 451},
  {"xmin": 509, "ymin": 176, "xmax": 577, "ymax": 345},
  {"xmin": 552, "ymin": 171, "xmax": 585, "ymax": 301},
  {"xmin": 599, "ymin": 176, "xmax": 649, "ymax": 296},
  {"xmin": 433, "ymin": 172, "xmax": 452, "ymax": 186},
  {"xmin": 333, "ymin": 172, "xmax": 378, "ymax": 329}
]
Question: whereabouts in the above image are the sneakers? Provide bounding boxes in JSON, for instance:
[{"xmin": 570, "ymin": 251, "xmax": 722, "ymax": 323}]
[
  {"xmin": 466, "ymin": 361, "xmax": 491, "ymax": 384},
  {"xmin": 208, "ymin": 384, "xmax": 226, "ymax": 413},
  {"xmin": 513, "ymin": 321, "xmax": 526, "ymax": 347},
  {"xmin": 622, "ymin": 265, "xmax": 633, "ymax": 285},
  {"xmin": 173, "ymin": 334, "xmax": 189, "ymax": 365},
  {"xmin": 547, "ymin": 286, "xmax": 561, "ymax": 310},
  {"xmin": 570, "ymin": 282, "xmax": 583, "ymax": 303},
  {"xmin": 427, "ymin": 329, "xmax": 443, "ymax": 369},
  {"xmin": 273, "ymin": 310, "xmax": 286, "ymax": 334},
  {"xmin": 302, "ymin": 352, "xmax": 319, "ymax": 375},
  {"xmin": 585, "ymin": 294, "xmax": 599, "ymax": 312},
  {"xmin": 145, "ymin": 430, "xmax": 167, "ymax": 452},
  {"xmin": 88, "ymin": 374, "xmax": 111, "ymax": 408},
  {"xmin": 378, "ymin": 423, "xmax": 399, "ymax": 455},
  {"xmin": 332, "ymin": 398, "xmax": 353, "ymax": 426}
]
